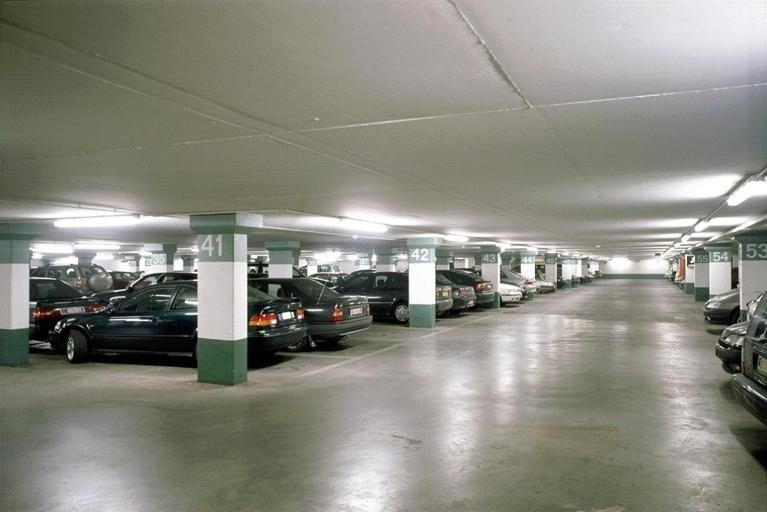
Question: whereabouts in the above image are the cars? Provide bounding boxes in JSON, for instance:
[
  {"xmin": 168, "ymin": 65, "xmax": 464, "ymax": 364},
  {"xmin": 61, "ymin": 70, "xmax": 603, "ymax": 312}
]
[
  {"xmin": 332, "ymin": 271, "xmax": 453, "ymax": 324},
  {"xmin": 48, "ymin": 278, "xmax": 308, "ymax": 364},
  {"xmin": 247, "ymin": 276, "xmax": 373, "ymax": 352},
  {"xmin": 474, "ymin": 264, "xmax": 602, "ymax": 306},
  {"xmin": 714, "ymin": 321, "xmax": 749, "ymax": 373},
  {"xmin": 404, "ymin": 269, "xmax": 475, "ymax": 318},
  {"xmin": 663, "ymin": 270, "xmax": 684, "ymax": 289},
  {"xmin": 30, "ymin": 262, "xmax": 197, "ymax": 342},
  {"xmin": 439, "ymin": 268, "xmax": 492, "ymax": 310},
  {"xmin": 731, "ymin": 267, "xmax": 739, "ymax": 286},
  {"xmin": 247, "ymin": 262, "xmax": 375, "ymax": 288},
  {"xmin": 702, "ymin": 287, "xmax": 740, "ymax": 326},
  {"xmin": 745, "ymin": 291, "xmax": 765, "ymax": 320},
  {"xmin": 337, "ymin": 268, "xmax": 375, "ymax": 284}
]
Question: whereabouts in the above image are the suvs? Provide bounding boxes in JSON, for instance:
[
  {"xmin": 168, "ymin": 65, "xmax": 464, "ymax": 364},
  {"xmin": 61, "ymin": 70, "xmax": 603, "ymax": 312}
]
[{"xmin": 731, "ymin": 290, "xmax": 767, "ymax": 424}]
